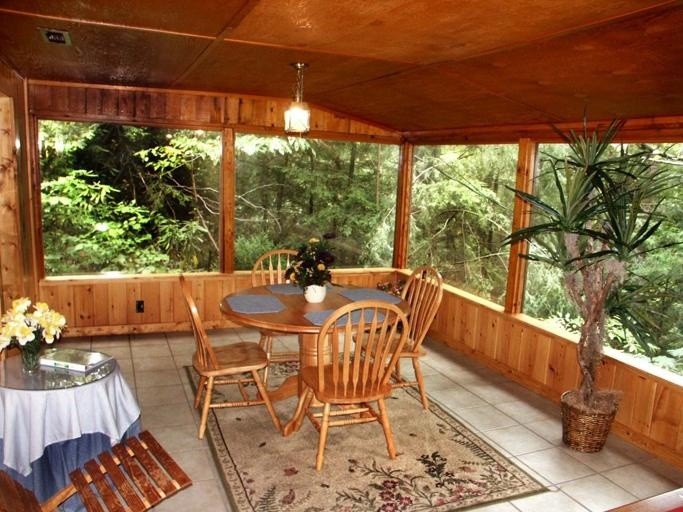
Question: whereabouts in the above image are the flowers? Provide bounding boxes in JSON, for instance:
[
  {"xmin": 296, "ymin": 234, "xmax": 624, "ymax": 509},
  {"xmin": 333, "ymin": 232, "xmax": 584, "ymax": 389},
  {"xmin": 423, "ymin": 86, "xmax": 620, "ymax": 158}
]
[
  {"xmin": 377, "ymin": 280, "xmax": 407, "ymax": 299},
  {"xmin": 0, "ymin": 296, "xmax": 66, "ymax": 371},
  {"xmin": 284, "ymin": 237, "xmax": 348, "ymax": 288}
]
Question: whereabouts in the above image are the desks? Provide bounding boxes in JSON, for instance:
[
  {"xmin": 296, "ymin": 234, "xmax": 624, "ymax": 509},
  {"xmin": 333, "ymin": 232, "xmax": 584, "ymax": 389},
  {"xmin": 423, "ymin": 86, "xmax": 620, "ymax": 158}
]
[{"xmin": 0, "ymin": 348, "xmax": 143, "ymax": 512}]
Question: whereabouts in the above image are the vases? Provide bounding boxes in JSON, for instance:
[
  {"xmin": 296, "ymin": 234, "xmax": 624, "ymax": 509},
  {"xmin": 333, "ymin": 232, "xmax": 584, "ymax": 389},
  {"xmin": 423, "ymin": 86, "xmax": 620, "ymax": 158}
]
[{"xmin": 304, "ymin": 284, "xmax": 327, "ymax": 303}]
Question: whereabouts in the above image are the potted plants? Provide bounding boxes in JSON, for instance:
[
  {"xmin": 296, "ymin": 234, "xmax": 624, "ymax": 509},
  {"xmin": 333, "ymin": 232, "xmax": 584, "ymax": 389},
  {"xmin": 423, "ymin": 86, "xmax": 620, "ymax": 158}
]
[{"xmin": 498, "ymin": 103, "xmax": 682, "ymax": 454}]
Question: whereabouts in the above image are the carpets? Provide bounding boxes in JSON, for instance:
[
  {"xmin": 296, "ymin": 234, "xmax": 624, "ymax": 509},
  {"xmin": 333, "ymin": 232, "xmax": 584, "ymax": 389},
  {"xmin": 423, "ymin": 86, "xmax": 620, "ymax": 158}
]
[{"xmin": 177, "ymin": 347, "xmax": 550, "ymax": 512}]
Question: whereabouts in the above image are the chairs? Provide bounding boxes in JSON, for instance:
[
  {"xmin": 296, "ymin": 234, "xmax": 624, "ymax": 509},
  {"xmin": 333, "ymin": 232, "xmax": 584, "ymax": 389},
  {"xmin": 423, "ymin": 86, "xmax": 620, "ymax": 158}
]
[
  {"xmin": 0, "ymin": 430, "xmax": 194, "ymax": 512},
  {"xmin": 351, "ymin": 265, "xmax": 444, "ymax": 411},
  {"xmin": 251, "ymin": 249, "xmax": 303, "ymax": 391},
  {"xmin": 294, "ymin": 299, "xmax": 409, "ymax": 472},
  {"xmin": 179, "ymin": 275, "xmax": 284, "ymax": 440}
]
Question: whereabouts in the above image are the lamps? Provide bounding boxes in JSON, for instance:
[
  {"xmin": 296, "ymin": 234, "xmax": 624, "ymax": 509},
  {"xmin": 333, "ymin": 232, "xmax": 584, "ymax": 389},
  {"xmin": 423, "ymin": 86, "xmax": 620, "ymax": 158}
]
[{"xmin": 284, "ymin": 62, "xmax": 312, "ymax": 134}]
